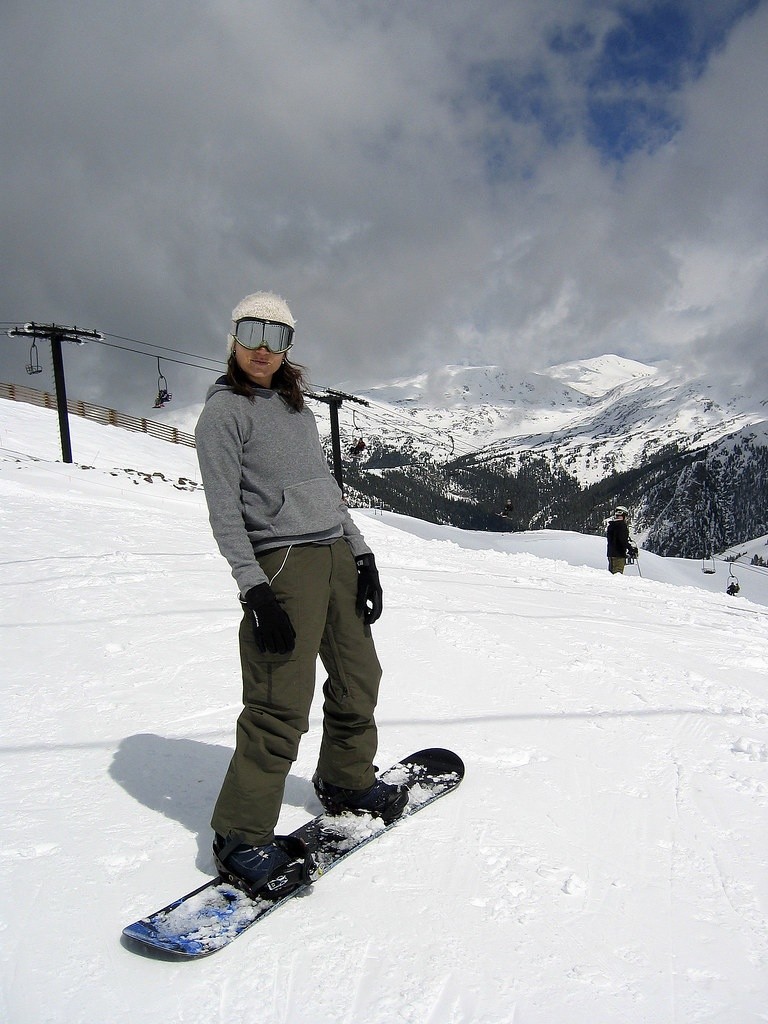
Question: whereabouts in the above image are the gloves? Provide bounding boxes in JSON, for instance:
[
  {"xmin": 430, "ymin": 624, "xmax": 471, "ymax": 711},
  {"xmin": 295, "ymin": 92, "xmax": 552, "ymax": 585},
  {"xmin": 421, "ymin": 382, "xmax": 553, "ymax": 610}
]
[
  {"xmin": 242, "ymin": 582, "xmax": 297, "ymax": 655},
  {"xmin": 354, "ymin": 553, "xmax": 382, "ymax": 623}
]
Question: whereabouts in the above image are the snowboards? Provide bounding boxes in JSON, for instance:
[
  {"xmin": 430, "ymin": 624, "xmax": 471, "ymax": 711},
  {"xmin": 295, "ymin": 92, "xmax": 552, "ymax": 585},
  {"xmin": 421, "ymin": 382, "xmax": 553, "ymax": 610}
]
[{"xmin": 119, "ymin": 747, "xmax": 467, "ymax": 962}]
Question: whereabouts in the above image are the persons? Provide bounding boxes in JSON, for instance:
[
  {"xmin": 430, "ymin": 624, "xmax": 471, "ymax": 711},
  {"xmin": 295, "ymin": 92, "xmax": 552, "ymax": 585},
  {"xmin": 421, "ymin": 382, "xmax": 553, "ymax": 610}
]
[
  {"xmin": 194, "ymin": 292, "xmax": 408, "ymax": 899},
  {"xmin": 606, "ymin": 506, "xmax": 637, "ymax": 575},
  {"xmin": 727, "ymin": 582, "xmax": 741, "ymax": 597},
  {"xmin": 153, "ymin": 390, "xmax": 169, "ymax": 408}
]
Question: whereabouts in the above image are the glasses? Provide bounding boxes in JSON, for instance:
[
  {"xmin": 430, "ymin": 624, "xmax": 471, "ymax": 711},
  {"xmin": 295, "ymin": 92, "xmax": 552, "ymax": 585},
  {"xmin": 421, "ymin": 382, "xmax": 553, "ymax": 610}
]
[{"xmin": 228, "ymin": 317, "xmax": 296, "ymax": 354}]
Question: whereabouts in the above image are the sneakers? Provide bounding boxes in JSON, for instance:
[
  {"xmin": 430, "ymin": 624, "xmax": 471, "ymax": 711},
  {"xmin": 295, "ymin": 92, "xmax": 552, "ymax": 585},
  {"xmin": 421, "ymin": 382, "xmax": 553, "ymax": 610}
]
[
  {"xmin": 212, "ymin": 831, "xmax": 303, "ymax": 896},
  {"xmin": 312, "ymin": 773, "xmax": 409, "ymax": 819}
]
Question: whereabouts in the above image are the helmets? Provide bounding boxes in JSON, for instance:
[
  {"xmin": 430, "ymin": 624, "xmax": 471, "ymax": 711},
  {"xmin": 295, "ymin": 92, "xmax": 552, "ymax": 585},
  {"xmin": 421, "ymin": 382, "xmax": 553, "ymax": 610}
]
[{"xmin": 615, "ymin": 506, "xmax": 628, "ymax": 516}]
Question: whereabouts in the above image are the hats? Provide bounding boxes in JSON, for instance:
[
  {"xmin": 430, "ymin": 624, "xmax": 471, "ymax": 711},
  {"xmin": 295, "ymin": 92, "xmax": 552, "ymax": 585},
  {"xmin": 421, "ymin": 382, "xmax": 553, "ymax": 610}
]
[{"xmin": 226, "ymin": 288, "xmax": 296, "ymax": 363}]
